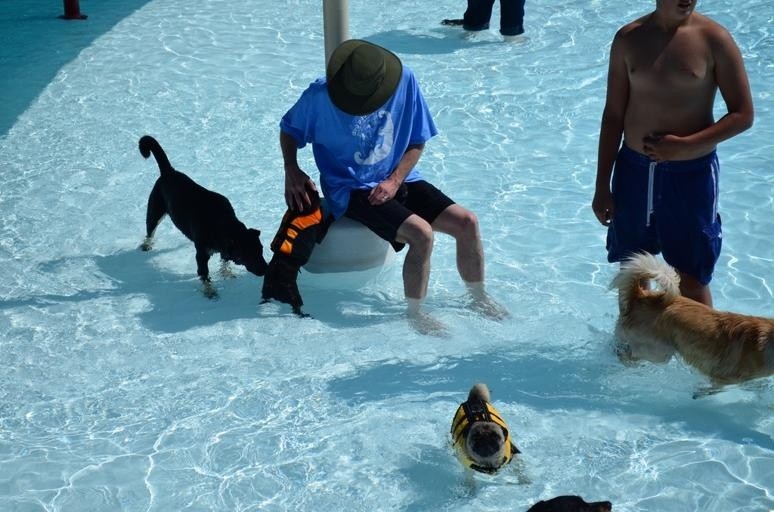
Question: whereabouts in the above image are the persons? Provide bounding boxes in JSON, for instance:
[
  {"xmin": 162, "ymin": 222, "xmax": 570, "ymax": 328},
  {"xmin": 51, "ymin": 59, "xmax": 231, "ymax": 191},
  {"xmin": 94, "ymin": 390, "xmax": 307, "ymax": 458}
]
[
  {"xmin": 439, "ymin": 0, "xmax": 534, "ymax": 47},
  {"xmin": 278, "ymin": 39, "xmax": 517, "ymax": 342},
  {"xmin": 590, "ymin": 0, "xmax": 756, "ymax": 314}
]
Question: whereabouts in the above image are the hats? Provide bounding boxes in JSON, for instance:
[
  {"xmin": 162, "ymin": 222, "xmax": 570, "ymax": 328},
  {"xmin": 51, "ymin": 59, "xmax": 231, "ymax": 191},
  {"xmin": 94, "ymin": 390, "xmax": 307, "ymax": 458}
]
[{"xmin": 327, "ymin": 39, "xmax": 402, "ymax": 114}]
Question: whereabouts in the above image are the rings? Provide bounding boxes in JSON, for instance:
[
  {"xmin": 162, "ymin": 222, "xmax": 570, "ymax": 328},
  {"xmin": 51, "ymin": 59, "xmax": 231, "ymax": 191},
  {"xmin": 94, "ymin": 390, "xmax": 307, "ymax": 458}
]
[{"xmin": 382, "ymin": 196, "xmax": 388, "ymax": 200}]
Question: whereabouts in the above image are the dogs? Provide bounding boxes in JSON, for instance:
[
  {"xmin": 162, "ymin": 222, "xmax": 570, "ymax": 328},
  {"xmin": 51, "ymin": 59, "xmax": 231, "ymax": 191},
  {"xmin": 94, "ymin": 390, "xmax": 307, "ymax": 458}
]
[
  {"xmin": 137, "ymin": 135, "xmax": 268, "ymax": 300},
  {"xmin": 452, "ymin": 383, "xmax": 533, "ymax": 498},
  {"xmin": 605, "ymin": 250, "xmax": 774, "ymax": 399},
  {"xmin": 259, "ymin": 184, "xmax": 336, "ymax": 319},
  {"xmin": 525, "ymin": 496, "xmax": 612, "ymax": 512}
]
